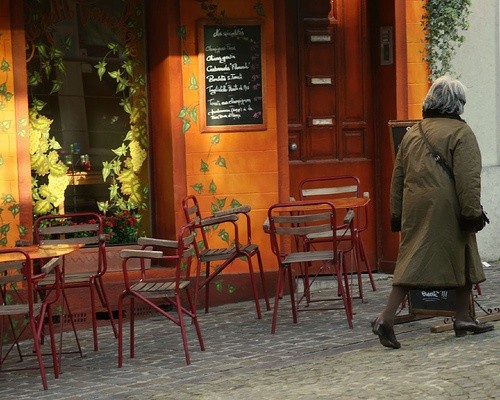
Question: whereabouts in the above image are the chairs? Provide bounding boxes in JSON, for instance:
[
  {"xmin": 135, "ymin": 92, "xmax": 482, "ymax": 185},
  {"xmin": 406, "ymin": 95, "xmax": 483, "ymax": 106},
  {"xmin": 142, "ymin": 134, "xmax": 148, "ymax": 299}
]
[{"xmin": 0, "ymin": 176, "xmax": 377, "ymax": 391}]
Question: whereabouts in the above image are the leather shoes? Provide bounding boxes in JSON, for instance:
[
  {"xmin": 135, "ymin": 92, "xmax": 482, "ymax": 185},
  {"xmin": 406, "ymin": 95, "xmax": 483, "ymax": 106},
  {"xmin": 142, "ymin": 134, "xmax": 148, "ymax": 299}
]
[
  {"xmin": 372, "ymin": 315, "xmax": 402, "ymax": 349},
  {"xmin": 454, "ymin": 320, "xmax": 494, "ymax": 337}
]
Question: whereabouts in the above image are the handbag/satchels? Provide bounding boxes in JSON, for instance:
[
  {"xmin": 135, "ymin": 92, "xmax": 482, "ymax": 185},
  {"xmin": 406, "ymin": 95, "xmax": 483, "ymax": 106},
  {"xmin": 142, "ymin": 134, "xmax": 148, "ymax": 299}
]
[{"xmin": 418, "ymin": 120, "xmax": 490, "ymax": 232}]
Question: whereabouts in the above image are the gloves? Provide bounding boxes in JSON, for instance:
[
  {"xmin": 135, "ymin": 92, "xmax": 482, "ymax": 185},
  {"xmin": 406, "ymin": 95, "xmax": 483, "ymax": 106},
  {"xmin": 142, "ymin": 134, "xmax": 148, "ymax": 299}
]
[
  {"xmin": 390, "ymin": 218, "xmax": 401, "ymax": 231},
  {"xmin": 459, "ymin": 218, "xmax": 483, "ymax": 231}
]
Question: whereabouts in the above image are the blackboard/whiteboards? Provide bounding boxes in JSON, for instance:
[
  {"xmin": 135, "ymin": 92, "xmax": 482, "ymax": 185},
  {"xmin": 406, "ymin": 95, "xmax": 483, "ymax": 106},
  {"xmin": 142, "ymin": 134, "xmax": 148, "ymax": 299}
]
[
  {"xmin": 393, "ymin": 126, "xmax": 471, "ymax": 313},
  {"xmin": 204, "ymin": 25, "xmax": 264, "ymax": 126}
]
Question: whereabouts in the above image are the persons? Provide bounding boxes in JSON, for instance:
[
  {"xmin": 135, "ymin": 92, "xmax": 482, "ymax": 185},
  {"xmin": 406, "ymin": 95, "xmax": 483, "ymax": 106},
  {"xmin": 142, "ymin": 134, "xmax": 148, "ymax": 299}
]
[{"xmin": 371, "ymin": 76, "xmax": 495, "ymax": 349}]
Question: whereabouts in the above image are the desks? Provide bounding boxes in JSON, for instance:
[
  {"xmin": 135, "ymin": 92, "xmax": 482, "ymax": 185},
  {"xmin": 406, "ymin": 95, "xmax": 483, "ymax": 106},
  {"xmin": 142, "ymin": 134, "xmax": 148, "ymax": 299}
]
[
  {"xmin": 66, "ymin": 170, "xmax": 113, "ymax": 186},
  {"xmin": 274, "ymin": 198, "xmax": 373, "ymax": 317},
  {"xmin": 0, "ymin": 243, "xmax": 85, "ymax": 372}
]
